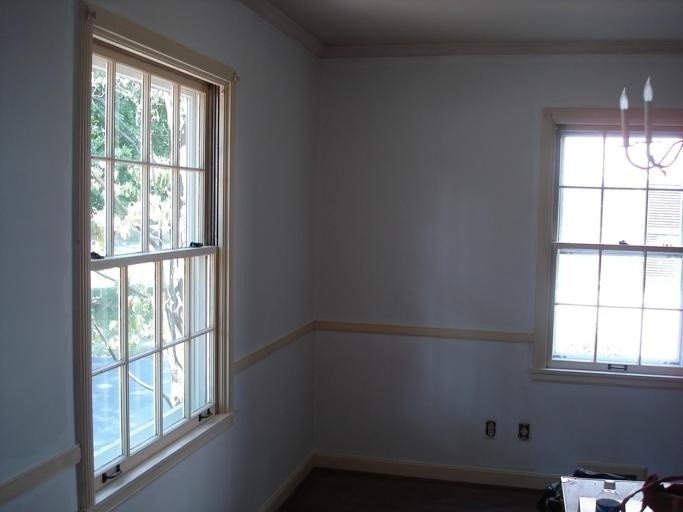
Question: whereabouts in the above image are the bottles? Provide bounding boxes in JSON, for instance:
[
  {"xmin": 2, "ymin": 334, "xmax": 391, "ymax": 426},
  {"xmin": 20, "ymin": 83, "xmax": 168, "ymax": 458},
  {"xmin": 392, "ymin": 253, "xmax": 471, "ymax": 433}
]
[{"xmin": 594, "ymin": 478, "xmax": 623, "ymax": 512}]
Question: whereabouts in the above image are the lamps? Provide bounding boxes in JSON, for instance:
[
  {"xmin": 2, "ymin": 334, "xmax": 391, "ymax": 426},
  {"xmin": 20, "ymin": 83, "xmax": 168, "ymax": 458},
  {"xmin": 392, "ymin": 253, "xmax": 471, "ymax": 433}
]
[{"xmin": 619, "ymin": 77, "xmax": 683, "ymax": 170}]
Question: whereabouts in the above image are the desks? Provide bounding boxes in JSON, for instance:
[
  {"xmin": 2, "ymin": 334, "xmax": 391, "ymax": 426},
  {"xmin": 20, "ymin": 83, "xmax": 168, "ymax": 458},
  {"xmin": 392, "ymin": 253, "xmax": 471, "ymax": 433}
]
[{"xmin": 560, "ymin": 476, "xmax": 683, "ymax": 511}]
